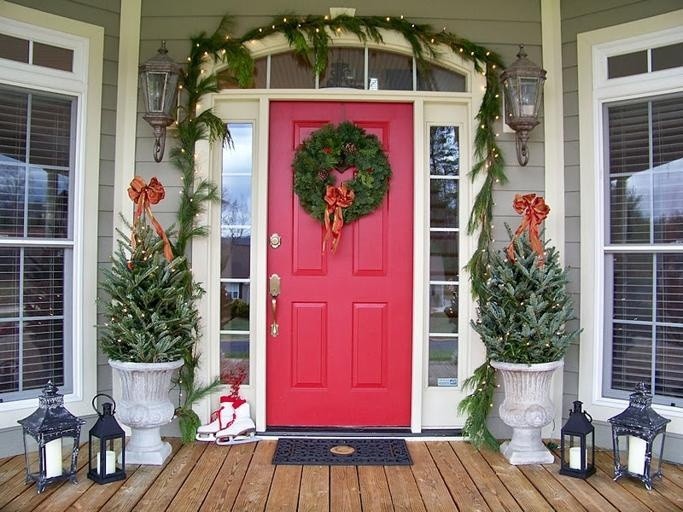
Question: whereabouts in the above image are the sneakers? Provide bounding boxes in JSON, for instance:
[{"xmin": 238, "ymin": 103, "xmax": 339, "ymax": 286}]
[
  {"xmin": 197, "ymin": 396, "xmax": 238, "ymax": 434},
  {"xmin": 216, "ymin": 400, "xmax": 256, "ymax": 438}
]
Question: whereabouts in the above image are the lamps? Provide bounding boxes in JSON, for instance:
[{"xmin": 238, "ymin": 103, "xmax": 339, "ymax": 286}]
[
  {"xmin": 139, "ymin": 40, "xmax": 182, "ymax": 162},
  {"xmin": 86, "ymin": 394, "xmax": 128, "ymax": 485},
  {"xmin": 608, "ymin": 381, "xmax": 672, "ymax": 490},
  {"xmin": 15, "ymin": 379, "xmax": 87, "ymax": 495},
  {"xmin": 500, "ymin": 43, "xmax": 547, "ymax": 167},
  {"xmin": 560, "ymin": 400, "xmax": 598, "ymax": 481}
]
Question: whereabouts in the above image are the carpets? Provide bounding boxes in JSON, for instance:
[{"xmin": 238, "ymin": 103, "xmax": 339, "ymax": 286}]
[{"xmin": 270, "ymin": 436, "xmax": 414, "ymax": 466}]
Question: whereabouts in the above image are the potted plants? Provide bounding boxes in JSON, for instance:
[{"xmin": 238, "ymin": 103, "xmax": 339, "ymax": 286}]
[
  {"xmin": 468, "ymin": 222, "xmax": 585, "ymax": 466},
  {"xmin": 93, "ymin": 204, "xmax": 205, "ymax": 466}
]
